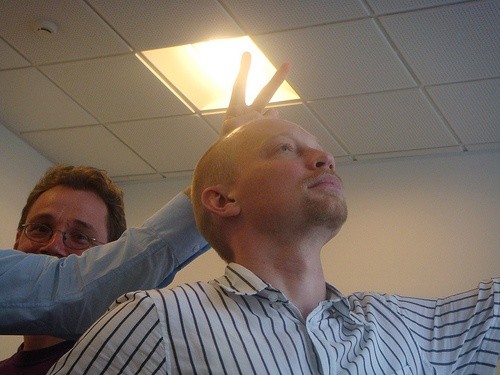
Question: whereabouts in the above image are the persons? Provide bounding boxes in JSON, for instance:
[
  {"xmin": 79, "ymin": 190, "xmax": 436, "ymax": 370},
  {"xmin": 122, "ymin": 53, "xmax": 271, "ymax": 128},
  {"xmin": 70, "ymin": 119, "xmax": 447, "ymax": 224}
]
[
  {"xmin": 0, "ymin": 50, "xmax": 291, "ymax": 375},
  {"xmin": 43, "ymin": 116, "xmax": 500, "ymax": 375},
  {"xmin": 0, "ymin": 162, "xmax": 127, "ymax": 375}
]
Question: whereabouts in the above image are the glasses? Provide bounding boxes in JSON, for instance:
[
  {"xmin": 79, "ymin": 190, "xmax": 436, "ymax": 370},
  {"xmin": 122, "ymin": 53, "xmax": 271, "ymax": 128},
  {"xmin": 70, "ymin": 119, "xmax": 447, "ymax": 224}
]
[{"xmin": 18, "ymin": 222, "xmax": 106, "ymax": 250}]
